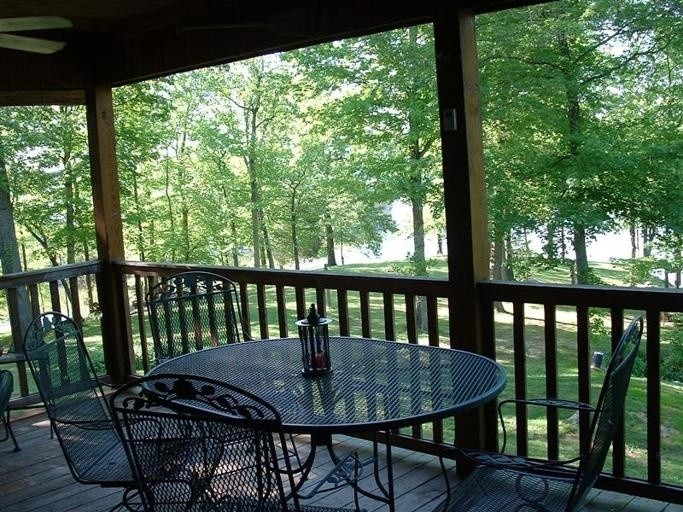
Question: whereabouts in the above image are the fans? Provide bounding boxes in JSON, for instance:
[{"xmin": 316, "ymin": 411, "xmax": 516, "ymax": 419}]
[{"xmin": 0, "ymin": 16, "xmax": 74, "ymax": 54}]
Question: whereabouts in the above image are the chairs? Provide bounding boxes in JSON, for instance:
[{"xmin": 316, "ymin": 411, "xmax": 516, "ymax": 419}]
[
  {"xmin": 23, "ymin": 311, "xmax": 224, "ymax": 512},
  {"xmin": 0, "ymin": 370, "xmax": 22, "ymax": 452},
  {"xmin": 110, "ymin": 374, "xmax": 367, "ymax": 512},
  {"xmin": 144, "ymin": 273, "xmax": 306, "ymax": 479},
  {"xmin": 431, "ymin": 316, "xmax": 644, "ymax": 512}
]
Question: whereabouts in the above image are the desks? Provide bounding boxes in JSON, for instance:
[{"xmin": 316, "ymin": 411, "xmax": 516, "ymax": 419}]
[{"xmin": 141, "ymin": 338, "xmax": 506, "ymax": 512}]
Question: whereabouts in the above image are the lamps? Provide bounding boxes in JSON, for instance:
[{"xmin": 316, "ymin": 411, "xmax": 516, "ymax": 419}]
[{"xmin": 295, "ymin": 303, "xmax": 333, "ymax": 376}]
